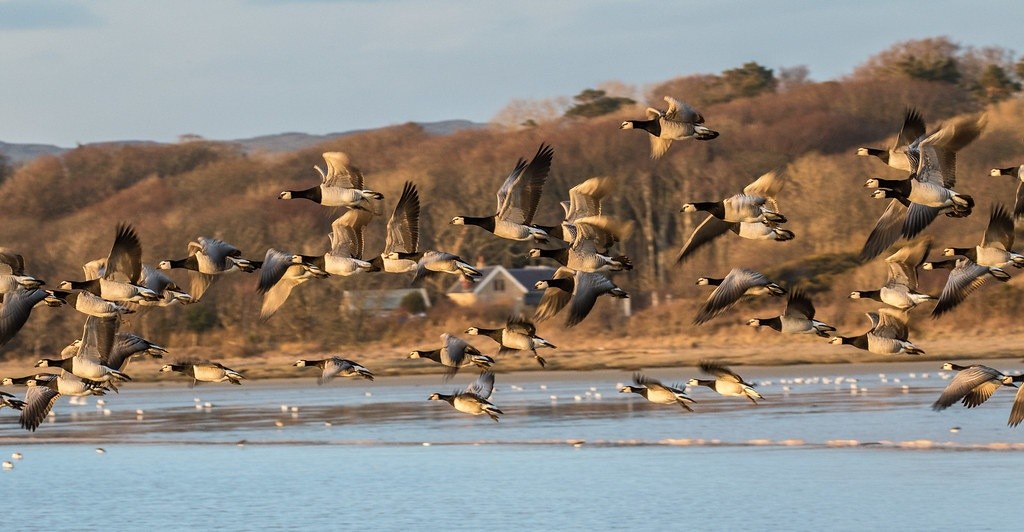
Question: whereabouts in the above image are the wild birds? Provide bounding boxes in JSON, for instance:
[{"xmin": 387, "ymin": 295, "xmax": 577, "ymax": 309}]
[
  {"xmin": 426, "ymin": 371, "xmax": 503, "ymax": 422},
  {"xmin": 406, "ymin": 333, "xmax": 493, "ymax": 373},
  {"xmin": 0, "ymin": 313, "xmax": 214, "ymax": 471},
  {"xmin": 493, "ymin": 385, "xmax": 603, "ymax": 403},
  {"xmin": 845, "ymin": 237, "xmax": 938, "ymax": 316},
  {"xmin": 761, "ymin": 373, "xmax": 950, "ymax": 395},
  {"xmin": 619, "ymin": 371, "xmax": 695, "ymax": 413},
  {"xmin": 294, "ymin": 357, "xmax": 376, "ymax": 386},
  {"xmin": 0, "ymin": 141, "xmax": 634, "ymax": 327},
  {"xmin": 826, "ymin": 308, "xmax": 926, "ymax": 356},
  {"xmin": 159, "ymin": 361, "xmax": 245, "ymax": 387},
  {"xmin": 618, "ymin": 96, "xmax": 718, "ymax": 162},
  {"xmin": 930, "ymin": 363, "xmax": 1024, "ymax": 429},
  {"xmin": 685, "ymin": 357, "xmax": 764, "ymax": 406},
  {"xmin": 236, "ymin": 392, "xmax": 430, "ymax": 448},
  {"xmin": 674, "ymin": 171, "xmax": 796, "ymax": 266},
  {"xmin": 851, "ymin": 107, "xmax": 990, "ymax": 262},
  {"xmin": 920, "ymin": 165, "xmax": 1024, "ymax": 321},
  {"xmin": 690, "ymin": 269, "xmax": 836, "ymax": 338},
  {"xmin": 464, "ymin": 316, "xmax": 557, "ymax": 372}
]
